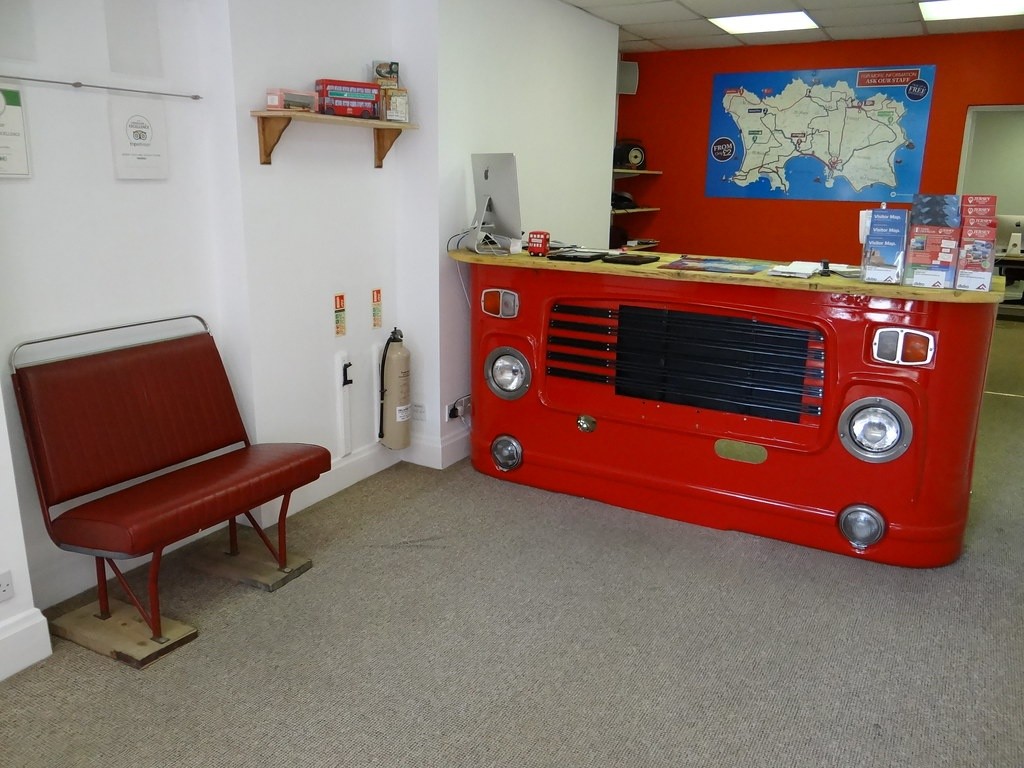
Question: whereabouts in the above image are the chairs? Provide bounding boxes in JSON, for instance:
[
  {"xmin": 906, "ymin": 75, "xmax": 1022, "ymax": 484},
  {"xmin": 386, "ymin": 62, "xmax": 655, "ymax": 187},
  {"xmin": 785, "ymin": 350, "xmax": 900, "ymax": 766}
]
[{"xmin": 996, "ymin": 215, "xmax": 1024, "ymax": 309}]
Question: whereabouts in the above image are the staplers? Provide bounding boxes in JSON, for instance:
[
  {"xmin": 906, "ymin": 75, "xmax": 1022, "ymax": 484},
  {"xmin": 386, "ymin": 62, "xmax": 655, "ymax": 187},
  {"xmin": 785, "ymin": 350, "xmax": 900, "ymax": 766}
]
[{"xmin": 818, "ymin": 260, "xmax": 832, "ymax": 277}]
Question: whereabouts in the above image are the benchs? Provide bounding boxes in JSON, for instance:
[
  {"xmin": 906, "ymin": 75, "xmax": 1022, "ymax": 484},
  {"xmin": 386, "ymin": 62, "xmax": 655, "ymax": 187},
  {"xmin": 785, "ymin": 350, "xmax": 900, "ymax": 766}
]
[{"xmin": 9, "ymin": 315, "xmax": 332, "ymax": 645}]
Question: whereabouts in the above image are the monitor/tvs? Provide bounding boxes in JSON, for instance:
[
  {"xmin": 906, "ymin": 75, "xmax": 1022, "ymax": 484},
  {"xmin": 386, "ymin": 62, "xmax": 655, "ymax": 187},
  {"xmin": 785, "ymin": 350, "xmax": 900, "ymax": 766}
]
[{"xmin": 464, "ymin": 153, "xmax": 522, "ymax": 254}]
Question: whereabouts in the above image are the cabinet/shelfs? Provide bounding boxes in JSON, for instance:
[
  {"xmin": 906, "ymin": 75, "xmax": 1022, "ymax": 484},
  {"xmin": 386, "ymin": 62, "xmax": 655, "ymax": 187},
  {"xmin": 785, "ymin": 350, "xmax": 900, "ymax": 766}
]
[{"xmin": 610, "ymin": 167, "xmax": 662, "ymax": 251}]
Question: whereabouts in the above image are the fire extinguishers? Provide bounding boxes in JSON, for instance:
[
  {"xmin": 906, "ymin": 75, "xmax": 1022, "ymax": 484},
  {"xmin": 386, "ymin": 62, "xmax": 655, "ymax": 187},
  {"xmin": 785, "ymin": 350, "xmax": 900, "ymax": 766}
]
[{"xmin": 378, "ymin": 326, "xmax": 411, "ymax": 449}]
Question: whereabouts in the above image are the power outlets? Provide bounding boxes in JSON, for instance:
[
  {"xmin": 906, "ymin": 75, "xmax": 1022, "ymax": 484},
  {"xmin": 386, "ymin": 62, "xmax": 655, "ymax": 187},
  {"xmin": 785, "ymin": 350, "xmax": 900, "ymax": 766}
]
[{"xmin": 445, "ymin": 396, "xmax": 470, "ymax": 423}]
